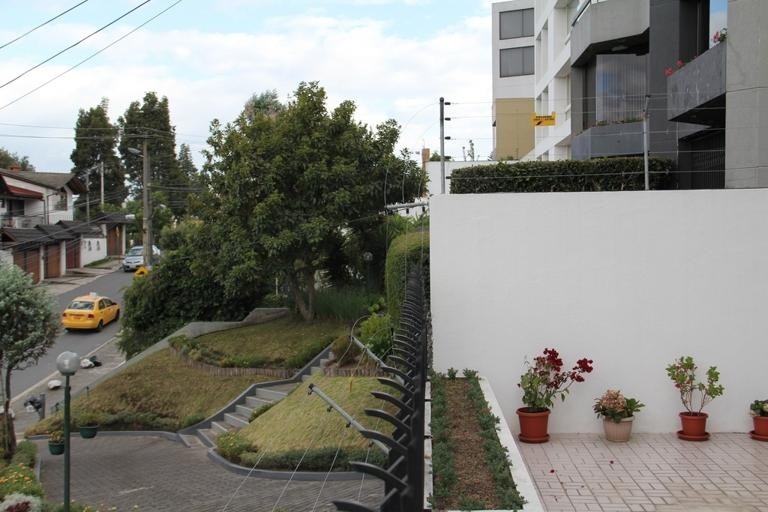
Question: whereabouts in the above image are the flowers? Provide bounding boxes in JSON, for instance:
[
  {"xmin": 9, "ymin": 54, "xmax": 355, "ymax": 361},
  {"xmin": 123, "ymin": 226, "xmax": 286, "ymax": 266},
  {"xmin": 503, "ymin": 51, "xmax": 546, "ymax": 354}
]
[
  {"xmin": 713, "ymin": 28, "xmax": 727, "ymax": 43},
  {"xmin": 676, "ymin": 60, "xmax": 685, "ymax": 68},
  {"xmin": 592, "ymin": 387, "xmax": 646, "ymax": 424},
  {"xmin": 749, "ymin": 399, "xmax": 768, "ymax": 417},
  {"xmin": 665, "ymin": 67, "xmax": 673, "ymax": 76},
  {"xmin": 517, "ymin": 348, "xmax": 593, "ymax": 412}
]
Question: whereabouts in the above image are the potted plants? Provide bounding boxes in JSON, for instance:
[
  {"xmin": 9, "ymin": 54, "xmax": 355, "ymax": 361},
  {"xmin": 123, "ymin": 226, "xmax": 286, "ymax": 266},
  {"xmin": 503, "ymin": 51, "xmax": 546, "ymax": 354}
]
[
  {"xmin": 80, "ymin": 412, "xmax": 99, "ymax": 438},
  {"xmin": 49, "ymin": 431, "xmax": 67, "ymax": 455},
  {"xmin": 665, "ymin": 355, "xmax": 725, "ymax": 441}
]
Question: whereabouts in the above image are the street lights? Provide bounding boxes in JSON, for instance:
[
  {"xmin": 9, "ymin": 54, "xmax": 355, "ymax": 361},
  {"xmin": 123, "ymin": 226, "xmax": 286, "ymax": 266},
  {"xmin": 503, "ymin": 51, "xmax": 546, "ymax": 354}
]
[
  {"xmin": 125, "ymin": 146, "xmax": 151, "ymax": 268},
  {"xmin": 55, "ymin": 350, "xmax": 78, "ymax": 512}
]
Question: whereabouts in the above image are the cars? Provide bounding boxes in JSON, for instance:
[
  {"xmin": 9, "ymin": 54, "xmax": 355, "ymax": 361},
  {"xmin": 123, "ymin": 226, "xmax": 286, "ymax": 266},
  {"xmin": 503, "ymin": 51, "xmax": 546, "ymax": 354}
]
[
  {"xmin": 122, "ymin": 244, "xmax": 160, "ymax": 272},
  {"xmin": 61, "ymin": 292, "xmax": 119, "ymax": 332}
]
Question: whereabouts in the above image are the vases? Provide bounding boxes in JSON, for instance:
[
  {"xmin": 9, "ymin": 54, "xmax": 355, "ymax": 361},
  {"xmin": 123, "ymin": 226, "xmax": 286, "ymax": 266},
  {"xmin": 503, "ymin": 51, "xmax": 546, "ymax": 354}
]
[
  {"xmin": 749, "ymin": 416, "xmax": 768, "ymax": 442},
  {"xmin": 516, "ymin": 408, "xmax": 552, "ymax": 443},
  {"xmin": 603, "ymin": 416, "xmax": 635, "ymax": 442}
]
